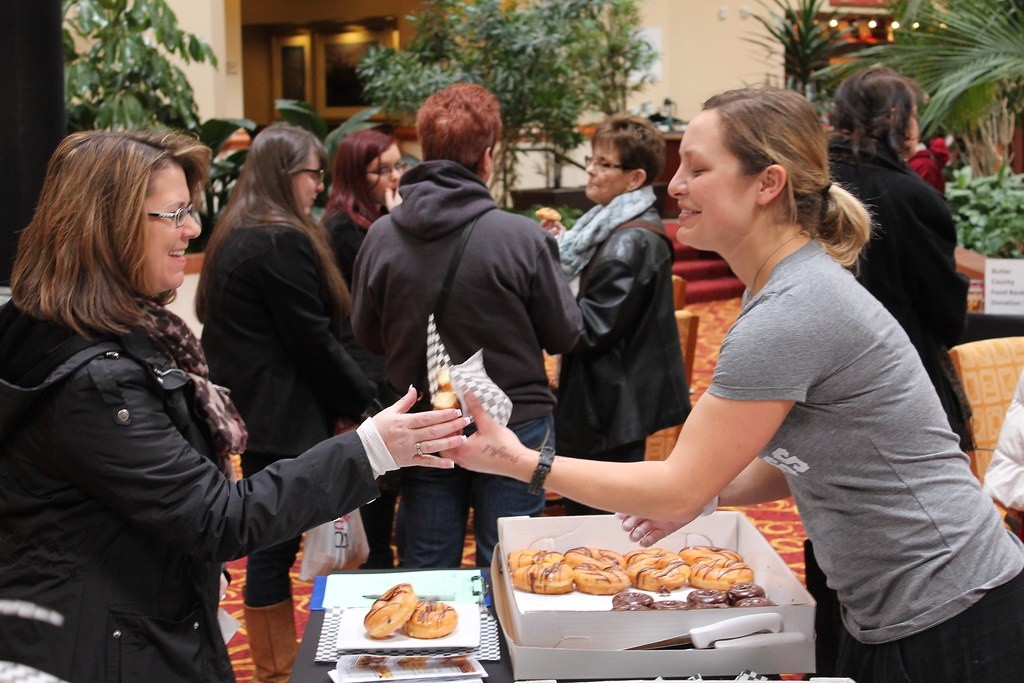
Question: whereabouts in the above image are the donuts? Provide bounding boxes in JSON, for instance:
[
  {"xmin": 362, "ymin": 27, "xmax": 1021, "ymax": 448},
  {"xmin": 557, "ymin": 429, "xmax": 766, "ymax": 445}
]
[
  {"xmin": 433, "ymin": 368, "xmax": 463, "ymax": 409},
  {"xmin": 404, "ymin": 602, "xmax": 458, "ymax": 638},
  {"xmin": 506, "ymin": 547, "xmax": 776, "ymax": 610},
  {"xmin": 364, "ymin": 584, "xmax": 417, "ymax": 638}
]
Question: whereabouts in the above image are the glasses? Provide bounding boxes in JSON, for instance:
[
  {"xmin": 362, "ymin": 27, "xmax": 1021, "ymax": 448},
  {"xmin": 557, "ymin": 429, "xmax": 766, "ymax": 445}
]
[
  {"xmin": 584, "ymin": 154, "xmax": 624, "ymax": 173},
  {"xmin": 292, "ymin": 168, "xmax": 325, "ymax": 184},
  {"xmin": 366, "ymin": 161, "xmax": 403, "ymax": 176},
  {"xmin": 145, "ymin": 204, "xmax": 195, "ymax": 227}
]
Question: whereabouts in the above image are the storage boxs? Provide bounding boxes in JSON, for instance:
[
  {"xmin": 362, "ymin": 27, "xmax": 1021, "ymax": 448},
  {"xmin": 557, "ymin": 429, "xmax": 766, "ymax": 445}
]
[{"xmin": 490, "ymin": 512, "xmax": 815, "ymax": 679}]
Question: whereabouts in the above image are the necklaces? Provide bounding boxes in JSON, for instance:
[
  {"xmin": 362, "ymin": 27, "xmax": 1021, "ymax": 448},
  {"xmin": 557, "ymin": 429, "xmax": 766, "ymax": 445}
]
[{"xmin": 750, "ymin": 229, "xmax": 805, "ymax": 300}]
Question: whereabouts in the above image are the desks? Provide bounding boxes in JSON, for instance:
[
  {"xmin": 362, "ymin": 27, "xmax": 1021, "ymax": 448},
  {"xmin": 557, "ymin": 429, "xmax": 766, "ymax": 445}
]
[{"xmin": 287, "ymin": 565, "xmax": 789, "ymax": 683}]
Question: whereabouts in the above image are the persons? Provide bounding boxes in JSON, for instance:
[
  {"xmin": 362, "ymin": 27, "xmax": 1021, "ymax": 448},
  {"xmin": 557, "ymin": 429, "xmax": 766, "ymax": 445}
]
[
  {"xmin": 0, "ymin": 128, "xmax": 471, "ymax": 683},
  {"xmin": 195, "ymin": 67, "xmax": 1024, "ymax": 683}
]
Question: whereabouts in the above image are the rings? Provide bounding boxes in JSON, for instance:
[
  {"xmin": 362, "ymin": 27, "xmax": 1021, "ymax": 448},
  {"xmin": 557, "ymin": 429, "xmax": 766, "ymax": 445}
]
[{"xmin": 415, "ymin": 442, "xmax": 424, "ymax": 457}]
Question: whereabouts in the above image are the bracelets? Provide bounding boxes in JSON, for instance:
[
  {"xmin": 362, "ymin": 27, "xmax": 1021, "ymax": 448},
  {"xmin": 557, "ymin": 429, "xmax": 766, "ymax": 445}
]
[{"xmin": 527, "ymin": 445, "xmax": 556, "ymax": 497}]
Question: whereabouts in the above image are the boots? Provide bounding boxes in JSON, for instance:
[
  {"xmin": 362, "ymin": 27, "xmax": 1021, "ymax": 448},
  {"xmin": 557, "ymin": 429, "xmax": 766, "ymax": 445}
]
[{"xmin": 241, "ymin": 586, "xmax": 303, "ymax": 682}]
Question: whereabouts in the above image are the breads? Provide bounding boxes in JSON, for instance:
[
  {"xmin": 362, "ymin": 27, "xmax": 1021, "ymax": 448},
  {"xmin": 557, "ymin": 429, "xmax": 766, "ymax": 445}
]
[{"xmin": 535, "ymin": 207, "xmax": 562, "ymax": 221}]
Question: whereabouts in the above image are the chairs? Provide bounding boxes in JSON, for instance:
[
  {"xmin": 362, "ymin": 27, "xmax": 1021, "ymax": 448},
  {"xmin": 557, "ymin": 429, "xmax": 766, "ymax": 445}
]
[
  {"xmin": 642, "ymin": 274, "xmax": 699, "ymax": 460},
  {"xmin": 952, "ymin": 337, "xmax": 1024, "ymax": 517}
]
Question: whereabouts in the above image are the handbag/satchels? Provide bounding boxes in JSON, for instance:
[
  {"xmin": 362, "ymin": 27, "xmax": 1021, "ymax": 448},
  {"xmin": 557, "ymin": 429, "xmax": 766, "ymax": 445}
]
[{"xmin": 300, "ymin": 508, "xmax": 370, "ymax": 582}]
